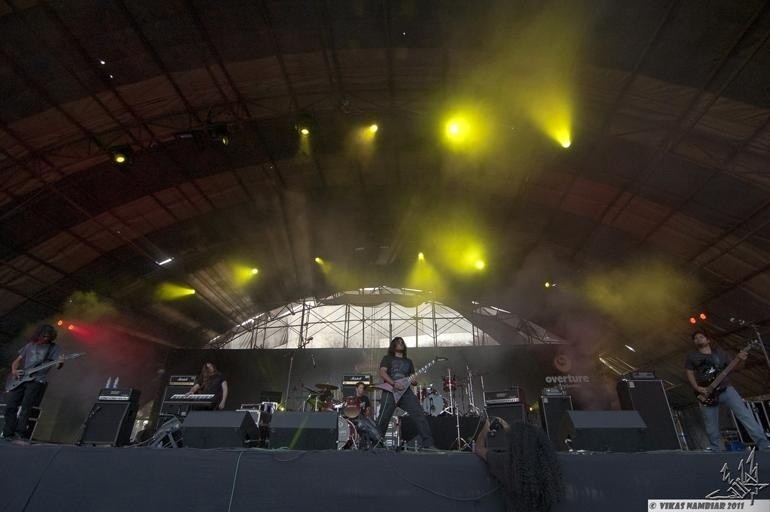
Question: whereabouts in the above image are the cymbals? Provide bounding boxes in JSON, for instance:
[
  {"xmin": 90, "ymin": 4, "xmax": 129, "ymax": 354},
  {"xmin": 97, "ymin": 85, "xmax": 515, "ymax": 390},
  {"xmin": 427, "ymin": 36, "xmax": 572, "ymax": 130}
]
[
  {"xmin": 315, "ymin": 384, "xmax": 339, "ymax": 390},
  {"xmin": 304, "ymin": 385, "xmax": 320, "ymax": 394}
]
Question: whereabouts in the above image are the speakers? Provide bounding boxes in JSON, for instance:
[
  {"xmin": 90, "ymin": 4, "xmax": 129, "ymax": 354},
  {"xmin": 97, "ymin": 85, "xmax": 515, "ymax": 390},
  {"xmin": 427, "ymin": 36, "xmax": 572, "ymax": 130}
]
[
  {"xmin": 617, "ymin": 380, "xmax": 683, "ymax": 451},
  {"xmin": 484, "ymin": 403, "xmax": 525, "ymax": 428},
  {"xmin": 181, "ymin": 411, "xmax": 261, "ymax": 449},
  {"xmin": 562, "ymin": 409, "xmax": 646, "ymax": 451},
  {"xmin": 79, "ymin": 401, "xmax": 138, "ymax": 447},
  {"xmin": 539, "ymin": 395, "xmax": 574, "ymax": 440},
  {"xmin": 268, "ymin": 412, "xmax": 338, "ymax": 449}
]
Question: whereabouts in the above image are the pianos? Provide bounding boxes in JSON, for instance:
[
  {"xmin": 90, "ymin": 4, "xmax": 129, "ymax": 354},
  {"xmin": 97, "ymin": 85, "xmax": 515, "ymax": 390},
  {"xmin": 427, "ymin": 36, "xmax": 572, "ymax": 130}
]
[{"xmin": 163, "ymin": 393, "xmax": 216, "ymax": 416}]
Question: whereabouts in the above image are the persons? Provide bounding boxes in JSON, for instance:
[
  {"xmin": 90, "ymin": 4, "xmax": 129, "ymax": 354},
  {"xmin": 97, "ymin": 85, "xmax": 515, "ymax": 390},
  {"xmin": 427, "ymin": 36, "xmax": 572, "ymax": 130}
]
[
  {"xmin": 372, "ymin": 337, "xmax": 435, "ymax": 449},
  {"xmin": 347, "ymin": 382, "xmax": 382, "ymax": 443},
  {"xmin": 0, "ymin": 324, "xmax": 64, "ymax": 441},
  {"xmin": 185, "ymin": 359, "xmax": 229, "ymax": 410},
  {"xmin": 685, "ymin": 331, "xmax": 770, "ymax": 452},
  {"xmin": 475, "ymin": 414, "xmax": 568, "ymax": 512}
]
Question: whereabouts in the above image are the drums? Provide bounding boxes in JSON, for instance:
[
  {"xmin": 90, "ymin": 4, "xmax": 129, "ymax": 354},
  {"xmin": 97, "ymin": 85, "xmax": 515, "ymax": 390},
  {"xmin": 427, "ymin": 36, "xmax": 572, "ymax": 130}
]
[
  {"xmin": 423, "ymin": 394, "xmax": 448, "ymax": 416},
  {"xmin": 442, "ymin": 397, "xmax": 454, "ymax": 404},
  {"xmin": 418, "ymin": 388, "xmax": 438, "ymax": 401},
  {"xmin": 342, "ymin": 396, "xmax": 361, "ymax": 418},
  {"xmin": 337, "ymin": 417, "xmax": 357, "ymax": 450},
  {"xmin": 443, "ymin": 376, "xmax": 457, "ymax": 392}
]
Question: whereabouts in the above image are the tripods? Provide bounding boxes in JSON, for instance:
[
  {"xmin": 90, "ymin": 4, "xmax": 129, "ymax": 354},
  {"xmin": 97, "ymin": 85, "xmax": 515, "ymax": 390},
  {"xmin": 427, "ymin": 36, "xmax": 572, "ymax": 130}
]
[{"xmin": 439, "ymin": 368, "xmax": 481, "ymax": 452}]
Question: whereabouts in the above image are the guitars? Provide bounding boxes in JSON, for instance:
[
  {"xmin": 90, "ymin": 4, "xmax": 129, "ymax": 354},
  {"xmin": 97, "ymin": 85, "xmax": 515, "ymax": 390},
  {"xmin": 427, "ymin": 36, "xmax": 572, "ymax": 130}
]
[
  {"xmin": 5, "ymin": 352, "xmax": 87, "ymax": 392},
  {"xmin": 694, "ymin": 344, "xmax": 752, "ymax": 408},
  {"xmin": 368, "ymin": 358, "xmax": 448, "ymax": 407}
]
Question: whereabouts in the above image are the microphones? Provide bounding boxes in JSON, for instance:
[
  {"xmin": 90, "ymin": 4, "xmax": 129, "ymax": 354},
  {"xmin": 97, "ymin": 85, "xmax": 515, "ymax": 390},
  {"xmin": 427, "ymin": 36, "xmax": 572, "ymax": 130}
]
[
  {"xmin": 312, "ymin": 355, "xmax": 316, "ymax": 368},
  {"xmin": 306, "ymin": 337, "xmax": 313, "ymax": 341}
]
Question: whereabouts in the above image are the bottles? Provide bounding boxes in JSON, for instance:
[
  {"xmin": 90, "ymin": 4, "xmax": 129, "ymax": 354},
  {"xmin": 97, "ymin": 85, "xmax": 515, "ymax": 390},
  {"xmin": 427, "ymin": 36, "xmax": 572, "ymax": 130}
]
[{"xmin": 106, "ymin": 377, "xmax": 121, "ymax": 389}]
